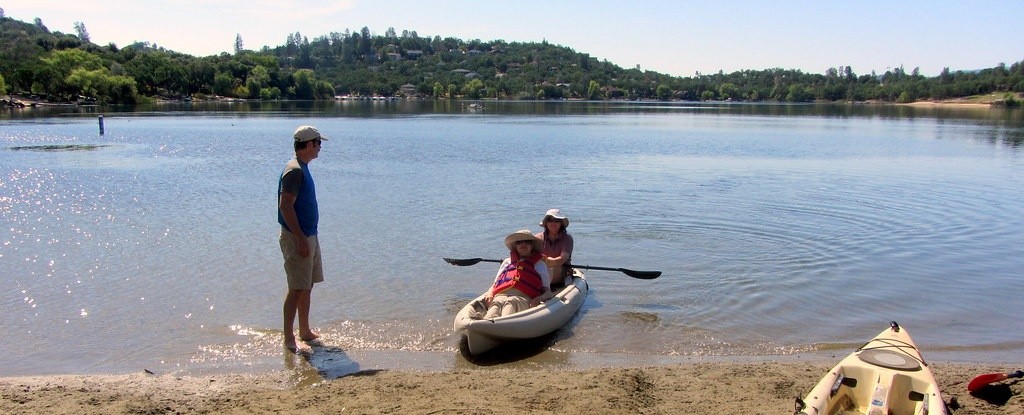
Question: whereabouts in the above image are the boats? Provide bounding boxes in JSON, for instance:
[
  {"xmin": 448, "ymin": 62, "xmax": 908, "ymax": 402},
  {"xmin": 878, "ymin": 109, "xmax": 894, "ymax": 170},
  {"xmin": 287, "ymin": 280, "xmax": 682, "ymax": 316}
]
[
  {"xmin": 794, "ymin": 319, "xmax": 949, "ymax": 415},
  {"xmin": 453, "ymin": 267, "xmax": 591, "ymax": 355}
]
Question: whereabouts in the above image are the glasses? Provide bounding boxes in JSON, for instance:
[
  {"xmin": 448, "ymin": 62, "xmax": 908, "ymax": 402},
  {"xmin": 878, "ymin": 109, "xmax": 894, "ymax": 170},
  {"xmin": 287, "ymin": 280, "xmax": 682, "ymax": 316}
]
[
  {"xmin": 546, "ymin": 217, "xmax": 562, "ymax": 223},
  {"xmin": 515, "ymin": 239, "xmax": 533, "ymax": 244}
]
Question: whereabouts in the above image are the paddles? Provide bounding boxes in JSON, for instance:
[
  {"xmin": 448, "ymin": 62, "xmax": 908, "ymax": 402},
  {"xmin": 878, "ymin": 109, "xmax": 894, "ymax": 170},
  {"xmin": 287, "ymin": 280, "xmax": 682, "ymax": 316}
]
[
  {"xmin": 966, "ymin": 370, "xmax": 1024, "ymax": 393},
  {"xmin": 441, "ymin": 255, "xmax": 663, "ymax": 281}
]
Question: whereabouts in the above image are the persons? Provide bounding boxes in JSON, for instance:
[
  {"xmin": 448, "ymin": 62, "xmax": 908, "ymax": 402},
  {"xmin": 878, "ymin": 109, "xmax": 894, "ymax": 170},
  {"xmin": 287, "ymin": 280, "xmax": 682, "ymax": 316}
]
[
  {"xmin": 534, "ymin": 209, "xmax": 573, "ymax": 291},
  {"xmin": 474, "ymin": 230, "xmax": 551, "ymax": 320},
  {"xmin": 278, "ymin": 125, "xmax": 329, "ymax": 347}
]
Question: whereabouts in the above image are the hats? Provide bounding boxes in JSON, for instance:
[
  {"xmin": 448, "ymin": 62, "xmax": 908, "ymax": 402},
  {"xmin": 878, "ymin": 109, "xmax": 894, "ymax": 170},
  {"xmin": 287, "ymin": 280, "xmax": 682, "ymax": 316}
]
[
  {"xmin": 539, "ymin": 209, "xmax": 569, "ymax": 228},
  {"xmin": 505, "ymin": 230, "xmax": 541, "ymax": 252},
  {"xmin": 293, "ymin": 126, "xmax": 328, "ymax": 142}
]
